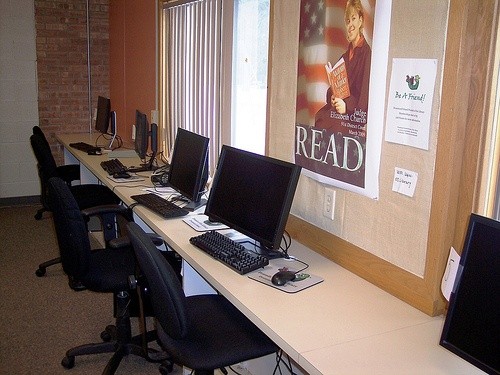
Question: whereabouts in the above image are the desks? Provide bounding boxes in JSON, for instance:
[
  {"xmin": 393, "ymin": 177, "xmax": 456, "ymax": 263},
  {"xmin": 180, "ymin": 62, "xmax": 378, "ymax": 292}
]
[{"xmin": 53, "ymin": 133, "xmax": 489, "ymax": 375}]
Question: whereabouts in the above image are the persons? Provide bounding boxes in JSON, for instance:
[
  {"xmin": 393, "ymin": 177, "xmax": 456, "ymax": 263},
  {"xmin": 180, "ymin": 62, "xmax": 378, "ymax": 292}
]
[{"xmin": 312, "ymin": 0, "xmax": 372, "ymax": 139}]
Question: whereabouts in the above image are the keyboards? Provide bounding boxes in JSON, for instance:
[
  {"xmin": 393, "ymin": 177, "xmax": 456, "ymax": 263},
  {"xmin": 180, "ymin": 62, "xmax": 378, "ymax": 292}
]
[
  {"xmin": 188, "ymin": 230, "xmax": 269, "ymax": 275},
  {"xmin": 100, "ymin": 158, "xmax": 128, "ymax": 175},
  {"xmin": 69, "ymin": 142, "xmax": 101, "ymax": 153},
  {"xmin": 130, "ymin": 193, "xmax": 189, "ymax": 218}
]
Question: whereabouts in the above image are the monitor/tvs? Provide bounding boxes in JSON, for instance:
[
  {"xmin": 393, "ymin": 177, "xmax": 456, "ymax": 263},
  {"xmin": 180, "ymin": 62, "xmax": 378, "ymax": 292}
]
[
  {"xmin": 94, "ymin": 96, "xmax": 157, "ymax": 172},
  {"xmin": 438, "ymin": 212, "xmax": 500, "ymax": 375},
  {"xmin": 167, "ymin": 127, "xmax": 210, "ymax": 212},
  {"xmin": 203, "ymin": 145, "xmax": 303, "ymax": 260}
]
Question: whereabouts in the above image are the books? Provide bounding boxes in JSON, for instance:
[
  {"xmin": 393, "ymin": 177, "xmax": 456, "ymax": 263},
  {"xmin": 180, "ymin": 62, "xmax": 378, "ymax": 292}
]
[{"xmin": 324, "ymin": 56, "xmax": 351, "ymax": 101}]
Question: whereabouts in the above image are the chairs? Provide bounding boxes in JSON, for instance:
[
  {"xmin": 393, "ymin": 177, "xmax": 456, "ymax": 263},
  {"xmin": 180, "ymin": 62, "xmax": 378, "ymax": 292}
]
[{"xmin": 30, "ymin": 126, "xmax": 281, "ymax": 375}]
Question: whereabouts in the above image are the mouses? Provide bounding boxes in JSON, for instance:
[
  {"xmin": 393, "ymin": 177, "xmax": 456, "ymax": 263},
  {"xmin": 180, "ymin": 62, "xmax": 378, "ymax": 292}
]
[
  {"xmin": 88, "ymin": 150, "xmax": 102, "ymax": 155},
  {"xmin": 113, "ymin": 173, "xmax": 131, "ymax": 179},
  {"xmin": 271, "ymin": 271, "xmax": 296, "ymax": 286}
]
